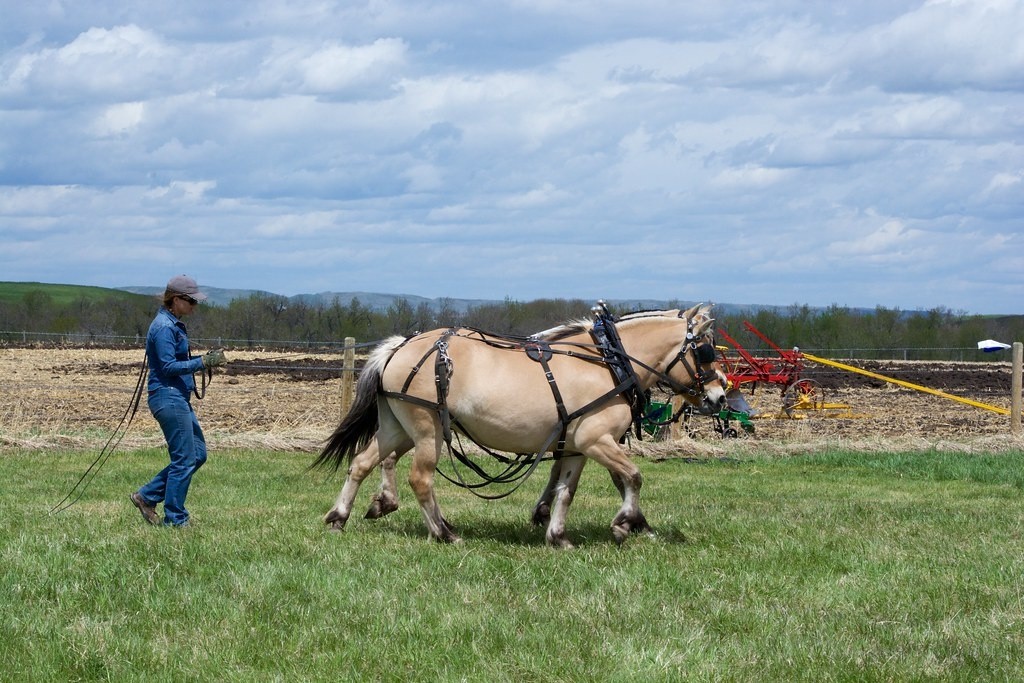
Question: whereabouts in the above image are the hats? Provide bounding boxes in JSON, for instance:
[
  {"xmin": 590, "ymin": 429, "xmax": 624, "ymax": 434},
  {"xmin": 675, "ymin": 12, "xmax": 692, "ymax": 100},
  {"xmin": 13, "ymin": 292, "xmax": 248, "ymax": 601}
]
[{"xmin": 168, "ymin": 274, "xmax": 208, "ymax": 301}]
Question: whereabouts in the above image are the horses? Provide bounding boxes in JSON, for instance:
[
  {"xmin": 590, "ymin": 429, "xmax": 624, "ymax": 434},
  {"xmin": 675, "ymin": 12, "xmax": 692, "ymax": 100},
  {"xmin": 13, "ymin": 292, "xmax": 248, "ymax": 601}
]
[{"xmin": 300, "ymin": 301, "xmax": 728, "ymax": 553}]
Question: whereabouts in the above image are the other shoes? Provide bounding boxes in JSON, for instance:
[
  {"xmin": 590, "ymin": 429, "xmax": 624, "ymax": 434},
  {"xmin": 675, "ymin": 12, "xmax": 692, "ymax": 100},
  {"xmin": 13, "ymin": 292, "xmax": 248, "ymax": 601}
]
[{"xmin": 130, "ymin": 491, "xmax": 160, "ymax": 526}]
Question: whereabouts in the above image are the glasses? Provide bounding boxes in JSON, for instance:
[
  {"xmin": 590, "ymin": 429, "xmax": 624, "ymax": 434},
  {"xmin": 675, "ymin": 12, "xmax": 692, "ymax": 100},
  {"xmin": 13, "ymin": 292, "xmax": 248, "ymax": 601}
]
[{"xmin": 177, "ymin": 296, "xmax": 197, "ymax": 306}]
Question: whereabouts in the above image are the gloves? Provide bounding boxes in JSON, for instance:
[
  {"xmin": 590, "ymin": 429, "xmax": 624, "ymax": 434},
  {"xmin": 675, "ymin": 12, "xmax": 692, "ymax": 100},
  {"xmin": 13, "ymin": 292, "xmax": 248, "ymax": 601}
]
[{"xmin": 203, "ymin": 349, "xmax": 227, "ymax": 368}]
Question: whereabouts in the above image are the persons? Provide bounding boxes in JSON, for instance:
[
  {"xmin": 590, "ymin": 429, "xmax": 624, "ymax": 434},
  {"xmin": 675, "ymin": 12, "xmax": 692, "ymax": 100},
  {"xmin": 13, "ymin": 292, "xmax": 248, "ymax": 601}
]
[{"xmin": 130, "ymin": 275, "xmax": 228, "ymax": 528}]
